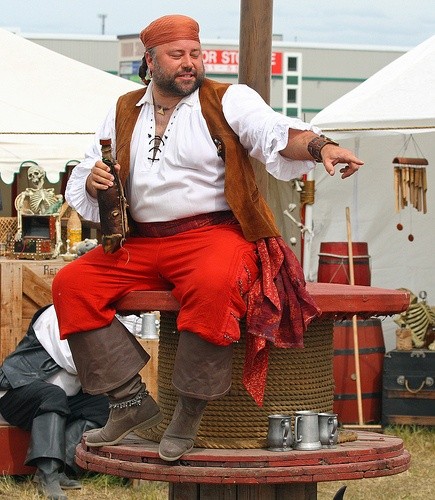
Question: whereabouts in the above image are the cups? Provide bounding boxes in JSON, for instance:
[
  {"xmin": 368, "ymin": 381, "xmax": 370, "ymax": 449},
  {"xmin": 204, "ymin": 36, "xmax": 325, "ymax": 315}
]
[
  {"xmin": 134, "ymin": 314, "xmax": 159, "ymax": 339},
  {"xmin": 266, "ymin": 415, "xmax": 294, "ymax": 452},
  {"xmin": 318, "ymin": 412, "xmax": 338, "ymax": 449},
  {"xmin": 293, "ymin": 411, "xmax": 321, "ymax": 451}
]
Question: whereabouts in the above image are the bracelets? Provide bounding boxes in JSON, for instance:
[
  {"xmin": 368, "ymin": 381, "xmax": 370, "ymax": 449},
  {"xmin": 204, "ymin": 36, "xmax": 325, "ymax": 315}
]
[{"xmin": 307, "ymin": 133, "xmax": 338, "ymax": 164}]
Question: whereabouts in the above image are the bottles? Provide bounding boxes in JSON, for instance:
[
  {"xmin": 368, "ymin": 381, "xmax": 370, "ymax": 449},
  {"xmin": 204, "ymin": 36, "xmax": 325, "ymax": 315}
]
[
  {"xmin": 396, "ymin": 324, "xmax": 412, "ymax": 351},
  {"xmin": 67, "ymin": 208, "xmax": 82, "ymax": 254},
  {"xmin": 96, "ymin": 138, "xmax": 129, "ymax": 238}
]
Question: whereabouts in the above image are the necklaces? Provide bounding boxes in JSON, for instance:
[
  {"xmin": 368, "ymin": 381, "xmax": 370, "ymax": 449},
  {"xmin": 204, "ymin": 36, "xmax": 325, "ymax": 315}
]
[{"xmin": 152, "ymin": 96, "xmax": 182, "ymax": 115}]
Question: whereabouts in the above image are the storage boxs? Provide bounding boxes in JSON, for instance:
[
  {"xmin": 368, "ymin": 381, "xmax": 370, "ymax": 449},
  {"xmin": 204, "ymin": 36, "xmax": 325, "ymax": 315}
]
[
  {"xmin": 12, "ymin": 213, "xmax": 57, "ymax": 258},
  {"xmin": 382, "ymin": 347, "xmax": 435, "ymax": 434}
]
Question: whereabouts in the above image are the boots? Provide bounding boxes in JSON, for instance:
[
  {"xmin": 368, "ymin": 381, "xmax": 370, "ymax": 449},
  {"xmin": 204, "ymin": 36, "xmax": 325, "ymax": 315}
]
[
  {"xmin": 34, "ymin": 418, "xmax": 100, "ymax": 490},
  {"xmin": 66, "ymin": 316, "xmax": 163, "ymax": 447},
  {"xmin": 24, "ymin": 412, "xmax": 66, "ymax": 500},
  {"xmin": 158, "ymin": 330, "xmax": 233, "ymax": 461}
]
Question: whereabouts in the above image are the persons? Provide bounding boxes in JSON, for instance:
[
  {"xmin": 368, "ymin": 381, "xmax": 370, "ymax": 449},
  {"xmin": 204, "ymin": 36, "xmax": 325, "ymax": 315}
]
[
  {"xmin": 53, "ymin": 15, "xmax": 366, "ymax": 461},
  {"xmin": 0, "ymin": 304, "xmax": 144, "ymax": 500}
]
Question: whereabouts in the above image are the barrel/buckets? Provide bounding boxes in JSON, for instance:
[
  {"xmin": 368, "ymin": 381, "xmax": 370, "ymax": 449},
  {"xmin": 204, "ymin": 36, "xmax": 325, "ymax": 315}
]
[
  {"xmin": 332, "ymin": 318, "xmax": 385, "ymax": 424},
  {"xmin": 317, "ymin": 242, "xmax": 371, "ymax": 319}
]
